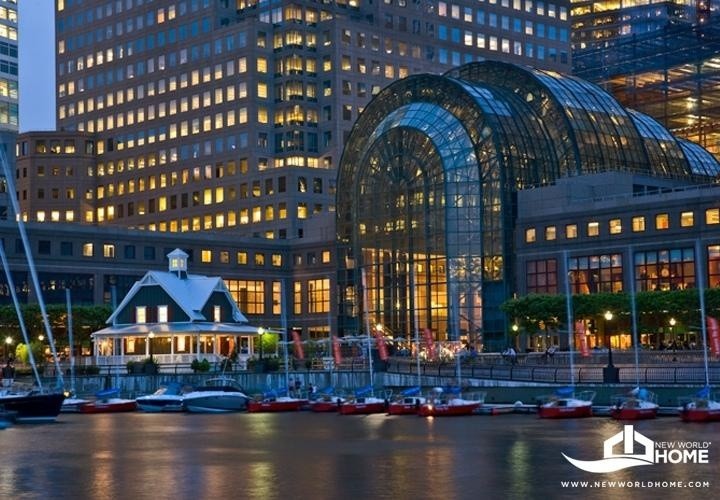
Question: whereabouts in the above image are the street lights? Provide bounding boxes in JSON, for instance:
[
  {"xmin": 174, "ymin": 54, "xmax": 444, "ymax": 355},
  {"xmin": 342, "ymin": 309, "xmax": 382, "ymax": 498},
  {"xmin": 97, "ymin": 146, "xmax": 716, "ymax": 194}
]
[
  {"xmin": 603, "ymin": 310, "xmax": 618, "ymax": 379},
  {"xmin": 256, "ymin": 326, "xmax": 267, "ymax": 361}
]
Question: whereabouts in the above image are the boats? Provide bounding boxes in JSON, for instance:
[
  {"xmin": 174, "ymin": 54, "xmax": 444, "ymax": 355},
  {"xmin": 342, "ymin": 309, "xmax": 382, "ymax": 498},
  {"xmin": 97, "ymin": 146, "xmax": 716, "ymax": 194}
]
[{"xmin": 0, "ymin": 379, "xmax": 718, "ymax": 428}]
